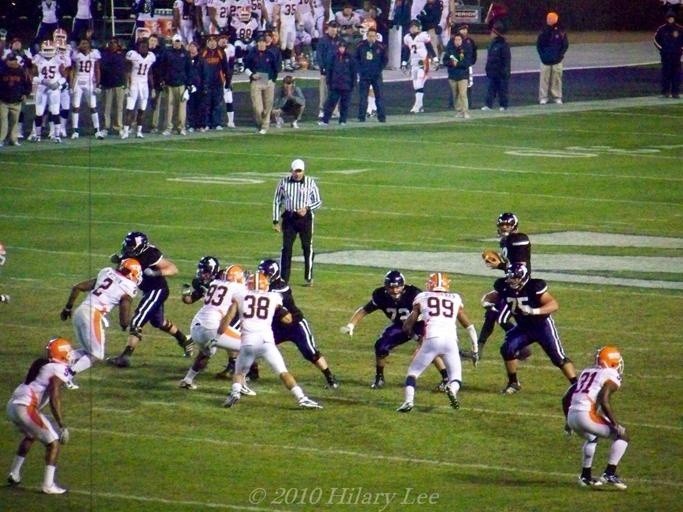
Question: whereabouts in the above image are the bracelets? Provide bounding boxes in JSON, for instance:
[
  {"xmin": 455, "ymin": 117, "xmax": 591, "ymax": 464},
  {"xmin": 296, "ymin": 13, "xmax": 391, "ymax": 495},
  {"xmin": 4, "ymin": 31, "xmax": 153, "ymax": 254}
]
[
  {"xmin": 1, "ymin": 295, "xmax": 6, "ymax": 302},
  {"xmin": 472, "ymin": 344, "xmax": 478, "ymax": 354},
  {"xmin": 65, "ymin": 302, "xmax": 74, "ymax": 310},
  {"xmin": 346, "ymin": 323, "xmax": 355, "ymax": 330},
  {"xmin": 613, "ymin": 422, "xmax": 626, "ymax": 436},
  {"xmin": 153, "ymin": 270, "xmax": 162, "ymax": 276},
  {"xmin": 412, "ymin": 334, "xmax": 419, "ymax": 341},
  {"xmin": 121, "ymin": 325, "xmax": 129, "ymax": 330}
]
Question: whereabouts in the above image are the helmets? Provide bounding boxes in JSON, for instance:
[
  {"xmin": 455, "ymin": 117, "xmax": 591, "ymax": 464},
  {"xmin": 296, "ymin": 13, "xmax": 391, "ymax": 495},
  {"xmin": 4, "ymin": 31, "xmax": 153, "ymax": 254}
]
[
  {"xmin": 497, "ymin": 211, "xmax": 517, "ymax": 238},
  {"xmin": 384, "ymin": 270, "xmax": 405, "ymax": 299},
  {"xmin": 596, "ymin": 348, "xmax": 624, "ymax": 375},
  {"xmin": 40, "ymin": 29, "xmax": 67, "ymax": 58},
  {"xmin": 427, "ymin": 272, "xmax": 450, "ymax": 292},
  {"xmin": 506, "ymin": 263, "xmax": 530, "ymax": 293},
  {"xmin": 46, "ymin": 338, "xmax": 75, "ymax": 367},
  {"xmin": 117, "ymin": 231, "xmax": 148, "ymax": 285},
  {"xmin": 197, "ymin": 256, "xmax": 280, "ymax": 290}
]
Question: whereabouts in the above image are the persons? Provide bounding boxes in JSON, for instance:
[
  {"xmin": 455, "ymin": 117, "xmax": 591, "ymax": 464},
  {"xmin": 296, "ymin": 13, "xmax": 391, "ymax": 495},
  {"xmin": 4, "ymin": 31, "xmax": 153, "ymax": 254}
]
[
  {"xmin": 178, "ymin": 256, "xmax": 337, "ymax": 411},
  {"xmin": 536, "ymin": 12, "xmax": 568, "ymax": 105},
  {"xmin": 653, "ymin": 12, "xmax": 683, "ymax": 99},
  {"xmin": 339, "ymin": 270, "xmax": 449, "ymax": 394},
  {"xmin": 104, "ymin": 231, "xmax": 195, "ymax": 368},
  {"xmin": 396, "ymin": 272, "xmax": 479, "ymax": 414},
  {"xmin": 561, "ymin": 346, "xmax": 630, "ymax": 490},
  {"xmin": 2, "ymin": 1, "xmax": 478, "ymax": 147},
  {"xmin": 0, "ymin": 243, "xmax": 11, "ymax": 304},
  {"xmin": 60, "ymin": 257, "xmax": 143, "ymax": 391},
  {"xmin": 272, "ymin": 159, "xmax": 321, "ymax": 288},
  {"xmin": 480, "ymin": 25, "xmax": 511, "ymax": 111},
  {"xmin": 460, "ymin": 213, "xmax": 578, "ymax": 396},
  {"xmin": 4, "ymin": 337, "xmax": 74, "ymax": 495}
]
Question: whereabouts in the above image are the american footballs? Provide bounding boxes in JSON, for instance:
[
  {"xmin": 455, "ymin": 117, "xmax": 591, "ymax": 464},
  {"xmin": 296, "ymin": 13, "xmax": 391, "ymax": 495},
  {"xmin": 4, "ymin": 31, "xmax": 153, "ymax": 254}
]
[{"xmin": 482, "ymin": 250, "xmax": 501, "ymax": 263}]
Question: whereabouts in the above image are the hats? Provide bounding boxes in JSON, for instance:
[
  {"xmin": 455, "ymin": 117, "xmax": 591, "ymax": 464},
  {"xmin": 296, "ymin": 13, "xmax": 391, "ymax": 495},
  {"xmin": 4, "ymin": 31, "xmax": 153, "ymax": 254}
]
[
  {"xmin": 492, "ymin": 23, "xmax": 503, "ymax": 36},
  {"xmin": 292, "ymin": 159, "xmax": 305, "ymax": 172},
  {"xmin": 172, "ymin": 34, "xmax": 181, "ymax": 42},
  {"xmin": 457, "ymin": 22, "xmax": 468, "ymax": 29}
]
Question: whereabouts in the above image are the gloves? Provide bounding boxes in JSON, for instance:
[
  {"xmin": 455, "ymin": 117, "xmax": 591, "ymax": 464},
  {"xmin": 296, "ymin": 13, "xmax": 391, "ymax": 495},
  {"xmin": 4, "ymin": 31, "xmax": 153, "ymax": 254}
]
[
  {"xmin": 483, "ymin": 303, "xmax": 498, "ymax": 313},
  {"xmin": 343, "ymin": 322, "xmax": 353, "ymax": 337},
  {"xmin": 1, "ymin": 294, "xmax": 9, "ymax": 304},
  {"xmin": 111, "ymin": 255, "xmax": 119, "ymax": 263},
  {"xmin": 61, "ymin": 304, "xmax": 71, "ymax": 322},
  {"xmin": 181, "ymin": 284, "xmax": 190, "ymax": 294},
  {"xmin": 412, "ymin": 334, "xmax": 421, "ymax": 341},
  {"xmin": 59, "ymin": 429, "xmax": 70, "ymax": 443},
  {"xmin": 145, "ymin": 267, "xmax": 162, "ymax": 277},
  {"xmin": 515, "ymin": 305, "xmax": 540, "ymax": 315},
  {"xmin": 615, "ymin": 424, "xmax": 625, "ymax": 435},
  {"xmin": 41, "ymin": 78, "xmax": 197, "ymax": 103},
  {"xmin": 564, "ymin": 422, "xmax": 573, "ymax": 440}
]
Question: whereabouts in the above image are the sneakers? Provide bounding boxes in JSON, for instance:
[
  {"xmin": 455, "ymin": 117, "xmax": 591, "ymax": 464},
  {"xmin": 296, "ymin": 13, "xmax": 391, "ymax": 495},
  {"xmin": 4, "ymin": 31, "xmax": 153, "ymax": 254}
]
[
  {"xmin": 107, "ymin": 356, "xmax": 131, "ymax": 366},
  {"xmin": 235, "ymin": 60, "xmax": 321, "ymax": 73},
  {"xmin": 7, "ymin": 473, "xmax": 21, "ymax": 483},
  {"xmin": 42, "ymin": 485, "xmax": 65, "ymax": 494},
  {"xmin": 578, "ymin": 476, "xmax": 603, "ymax": 486},
  {"xmin": 179, "ymin": 334, "xmax": 339, "ymax": 408},
  {"xmin": 601, "ymin": 473, "xmax": 627, "ymax": 490},
  {"xmin": 371, "ymin": 349, "xmax": 532, "ymax": 411},
  {"xmin": 410, "ymin": 98, "xmax": 563, "ymax": 119},
  {"xmin": 65, "ymin": 382, "xmax": 79, "ymax": 390}
]
